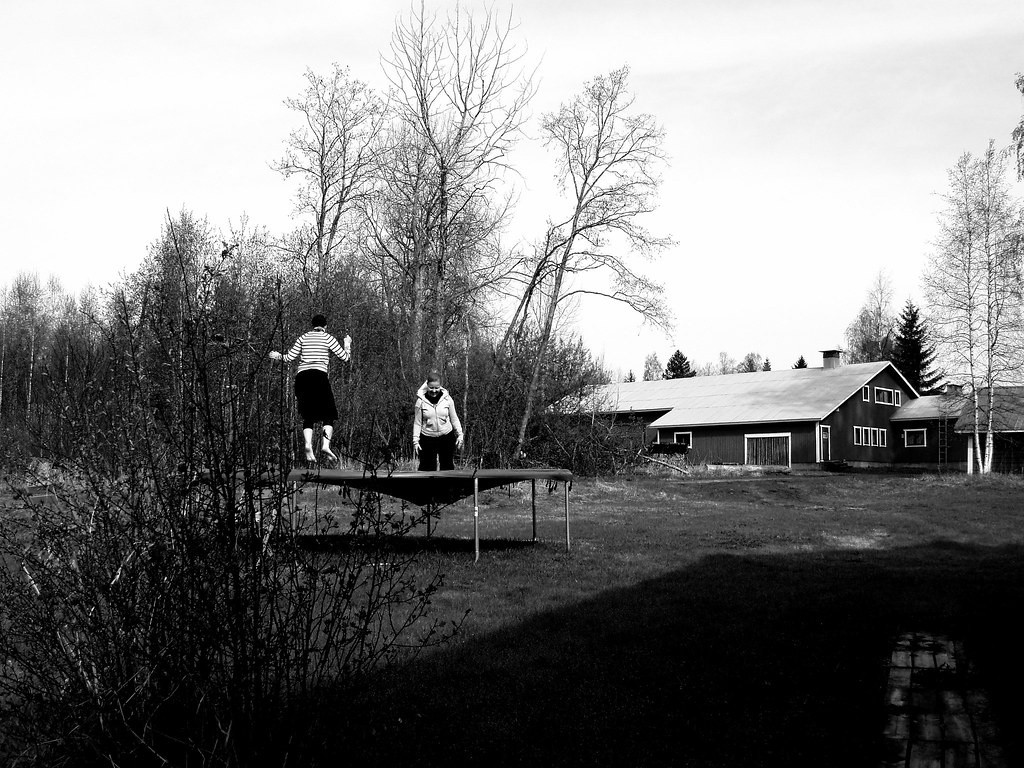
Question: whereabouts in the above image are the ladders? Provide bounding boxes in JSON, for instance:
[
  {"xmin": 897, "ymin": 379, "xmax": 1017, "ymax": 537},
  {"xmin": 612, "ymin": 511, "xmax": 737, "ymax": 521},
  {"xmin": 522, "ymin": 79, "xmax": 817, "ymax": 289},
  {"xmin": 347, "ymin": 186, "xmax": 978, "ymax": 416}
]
[{"xmin": 939, "ymin": 403, "xmax": 947, "ymax": 465}]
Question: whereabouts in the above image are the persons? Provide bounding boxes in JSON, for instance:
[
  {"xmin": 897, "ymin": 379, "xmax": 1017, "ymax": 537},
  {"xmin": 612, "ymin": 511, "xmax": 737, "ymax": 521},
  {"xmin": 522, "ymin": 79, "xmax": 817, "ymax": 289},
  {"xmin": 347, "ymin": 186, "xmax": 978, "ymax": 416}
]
[
  {"xmin": 269, "ymin": 315, "xmax": 352, "ymax": 462},
  {"xmin": 412, "ymin": 373, "xmax": 464, "ymax": 471}
]
